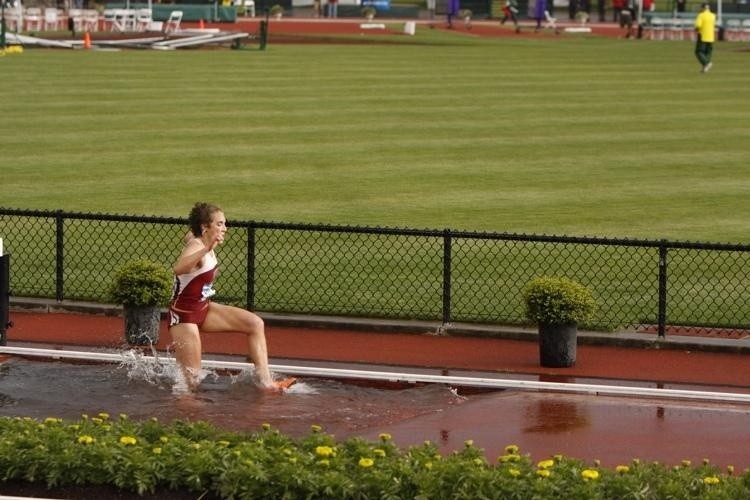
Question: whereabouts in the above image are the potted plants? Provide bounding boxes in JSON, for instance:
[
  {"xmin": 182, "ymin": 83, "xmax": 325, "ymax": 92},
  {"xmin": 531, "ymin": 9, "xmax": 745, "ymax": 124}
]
[
  {"xmin": 107, "ymin": 258, "xmax": 175, "ymax": 346},
  {"xmin": 522, "ymin": 276, "xmax": 596, "ymax": 367}
]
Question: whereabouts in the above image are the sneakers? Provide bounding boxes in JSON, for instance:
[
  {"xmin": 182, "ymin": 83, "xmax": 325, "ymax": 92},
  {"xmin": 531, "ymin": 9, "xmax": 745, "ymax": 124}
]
[
  {"xmin": 703, "ymin": 63, "xmax": 712, "ymax": 73},
  {"xmin": 275, "ymin": 377, "xmax": 296, "ymax": 389}
]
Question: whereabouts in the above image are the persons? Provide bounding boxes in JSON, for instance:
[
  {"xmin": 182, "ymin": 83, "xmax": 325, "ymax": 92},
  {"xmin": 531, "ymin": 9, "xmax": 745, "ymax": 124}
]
[
  {"xmin": 313, "ymin": 1, "xmax": 325, "ymax": 19},
  {"xmin": 567, "ymin": 1, "xmax": 688, "ymax": 24},
  {"xmin": 166, "ymin": 201, "xmax": 298, "ymax": 408},
  {"xmin": 326, "ymin": 0, "xmax": 338, "ymax": 20},
  {"xmin": 693, "ymin": 2, "xmax": 718, "ymax": 73},
  {"xmin": 497, "ymin": 0, "xmax": 523, "ymax": 34},
  {"xmin": 445, "ymin": 1, "xmax": 461, "ymax": 30},
  {"xmin": 427, "ymin": 0, "xmax": 436, "ymax": 21},
  {"xmin": 616, "ymin": 0, "xmax": 637, "ymax": 40},
  {"xmin": 533, "ymin": 0, "xmax": 548, "ymax": 33}
]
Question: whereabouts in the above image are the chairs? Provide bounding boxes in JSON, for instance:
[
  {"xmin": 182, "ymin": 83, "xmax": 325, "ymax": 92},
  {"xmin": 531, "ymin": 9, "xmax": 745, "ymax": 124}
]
[
  {"xmin": 5, "ymin": 2, "xmax": 184, "ymax": 36},
  {"xmin": 641, "ymin": 17, "xmax": 750, "ymax": 42}
]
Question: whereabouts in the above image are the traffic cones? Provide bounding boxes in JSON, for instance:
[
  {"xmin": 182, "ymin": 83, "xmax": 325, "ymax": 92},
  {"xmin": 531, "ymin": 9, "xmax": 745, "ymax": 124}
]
[
  {"xmin": 198, "ymin": 19, "xmax": 205, "ymax": 29},
  {"xmin": 81, "ymin": 29, "xmax": 94, "ymax": 48}
]
